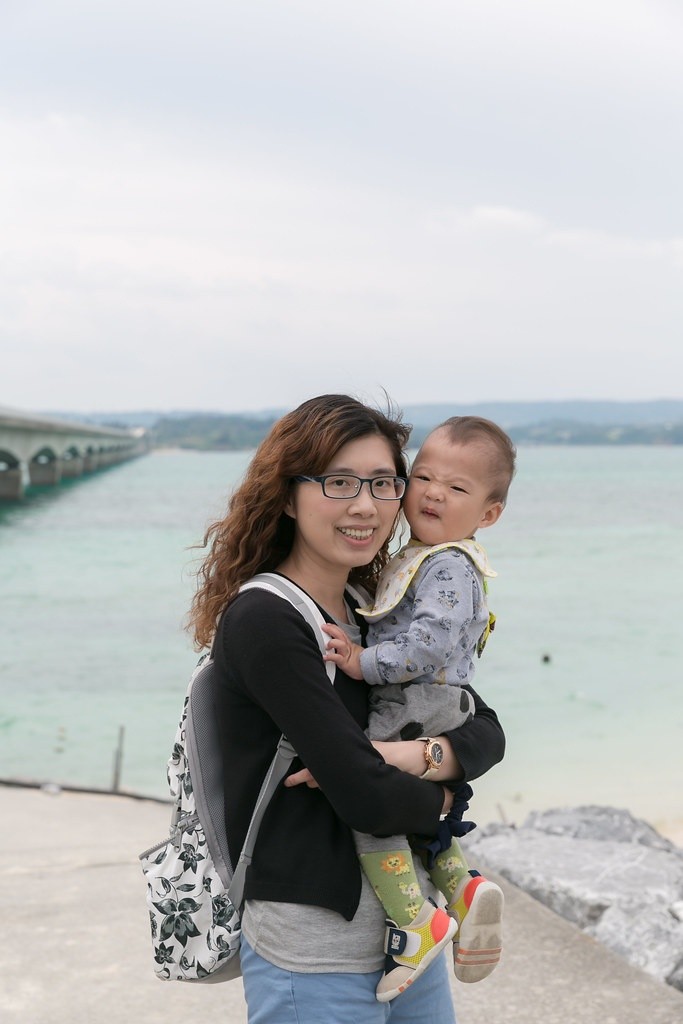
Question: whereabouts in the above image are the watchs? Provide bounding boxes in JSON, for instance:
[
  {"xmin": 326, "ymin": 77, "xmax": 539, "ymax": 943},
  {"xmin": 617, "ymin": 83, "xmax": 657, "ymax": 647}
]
[{"xmin": 415, "ymin": 737, "xmax": 446, "ymax": 781}]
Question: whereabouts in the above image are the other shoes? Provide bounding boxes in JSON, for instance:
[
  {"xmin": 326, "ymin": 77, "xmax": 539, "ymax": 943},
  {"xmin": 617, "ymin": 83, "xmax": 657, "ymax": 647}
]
[
  {"xmin": 450, "ymin": 869, "xmax": 504, "ymax": 984},
  {"xmin": 375, "ymin": 900, "xmax": 459, "ymax": 1004}
]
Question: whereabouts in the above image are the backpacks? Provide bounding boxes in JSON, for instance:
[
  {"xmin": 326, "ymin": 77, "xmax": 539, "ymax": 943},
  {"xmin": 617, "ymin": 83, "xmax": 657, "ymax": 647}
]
[{"xmin": 139, "ymin": 570, "xmax": 337, "ymax": 984}]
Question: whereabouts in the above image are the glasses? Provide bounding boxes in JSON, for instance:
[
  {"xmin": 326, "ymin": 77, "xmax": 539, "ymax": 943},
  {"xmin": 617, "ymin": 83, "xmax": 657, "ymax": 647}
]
[{"xmin": 304, "ymin": 471, "xmax": 406, "ymax": 500}]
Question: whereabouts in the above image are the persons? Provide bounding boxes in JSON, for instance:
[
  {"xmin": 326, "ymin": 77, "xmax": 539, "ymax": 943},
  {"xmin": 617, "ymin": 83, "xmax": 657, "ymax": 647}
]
[
  {"xmin": 323, "ymin": 416, "xmax": 518, "ymax": 1002},
  {"xmin": 221, "ymin": 393, "xmax": 506, "ymax": 1024}
]
